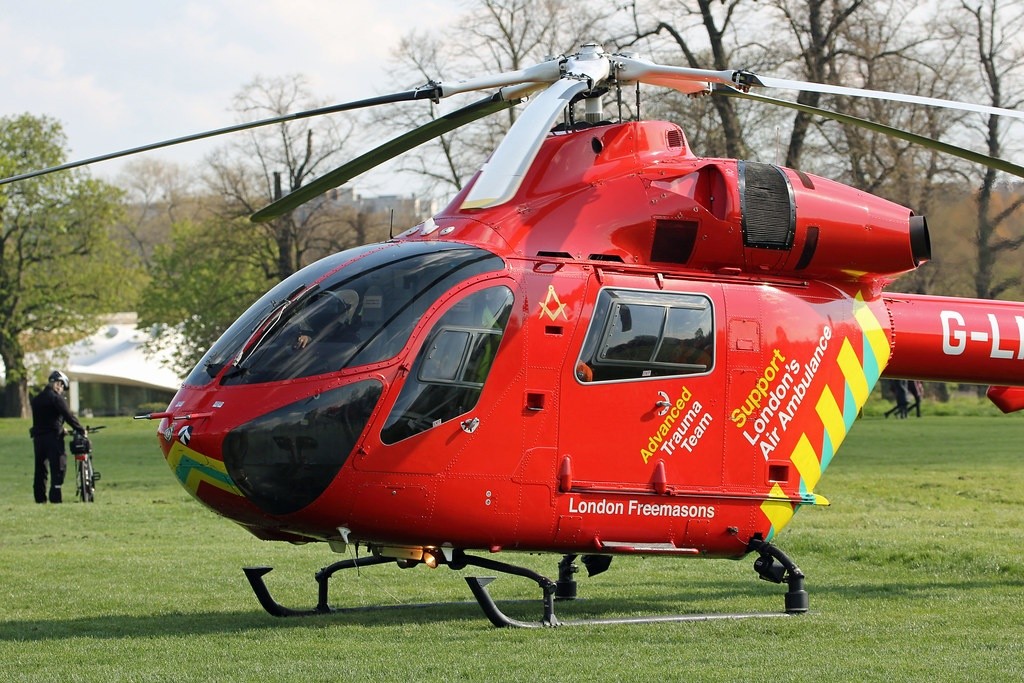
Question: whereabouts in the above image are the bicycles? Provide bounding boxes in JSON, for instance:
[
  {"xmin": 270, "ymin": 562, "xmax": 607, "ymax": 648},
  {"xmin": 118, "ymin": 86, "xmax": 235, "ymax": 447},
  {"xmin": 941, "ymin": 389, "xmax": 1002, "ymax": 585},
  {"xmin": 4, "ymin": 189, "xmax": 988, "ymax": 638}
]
[{"xmin": 59, "ymin": 425, "xmax": 106, "ymax": 504}]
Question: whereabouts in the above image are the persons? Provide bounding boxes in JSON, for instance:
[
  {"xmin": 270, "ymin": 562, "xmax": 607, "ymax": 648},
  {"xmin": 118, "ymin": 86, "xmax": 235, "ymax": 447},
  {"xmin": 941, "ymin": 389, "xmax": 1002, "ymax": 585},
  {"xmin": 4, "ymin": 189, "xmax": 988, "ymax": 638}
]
[
  {"xmin": 30, "ymin": 371, "xmax": 88, "ymax": 504},
  {"xmin": 292, "ymin": 291, "xmax": 370, "ymax": 349},
  {"xmin": 884, "ymin": 380, "xmax": 924, "ymax": 419}
]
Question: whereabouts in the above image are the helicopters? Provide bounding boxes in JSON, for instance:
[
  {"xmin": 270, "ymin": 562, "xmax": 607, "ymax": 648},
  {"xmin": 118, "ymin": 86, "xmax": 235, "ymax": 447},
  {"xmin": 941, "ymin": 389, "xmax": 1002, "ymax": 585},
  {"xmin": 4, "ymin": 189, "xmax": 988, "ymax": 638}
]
[{"xmin": 0, "ymin": 42, "xmax": 1024, "ymax": 630}]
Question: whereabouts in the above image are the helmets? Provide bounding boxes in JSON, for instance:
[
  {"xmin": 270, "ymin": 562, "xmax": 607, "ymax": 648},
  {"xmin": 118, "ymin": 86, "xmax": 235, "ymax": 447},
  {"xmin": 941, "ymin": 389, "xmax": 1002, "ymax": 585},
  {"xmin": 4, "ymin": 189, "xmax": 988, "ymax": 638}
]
[{"xmin": 50, "ymin": 371, "xmax": 69, "ymax": 391}]
